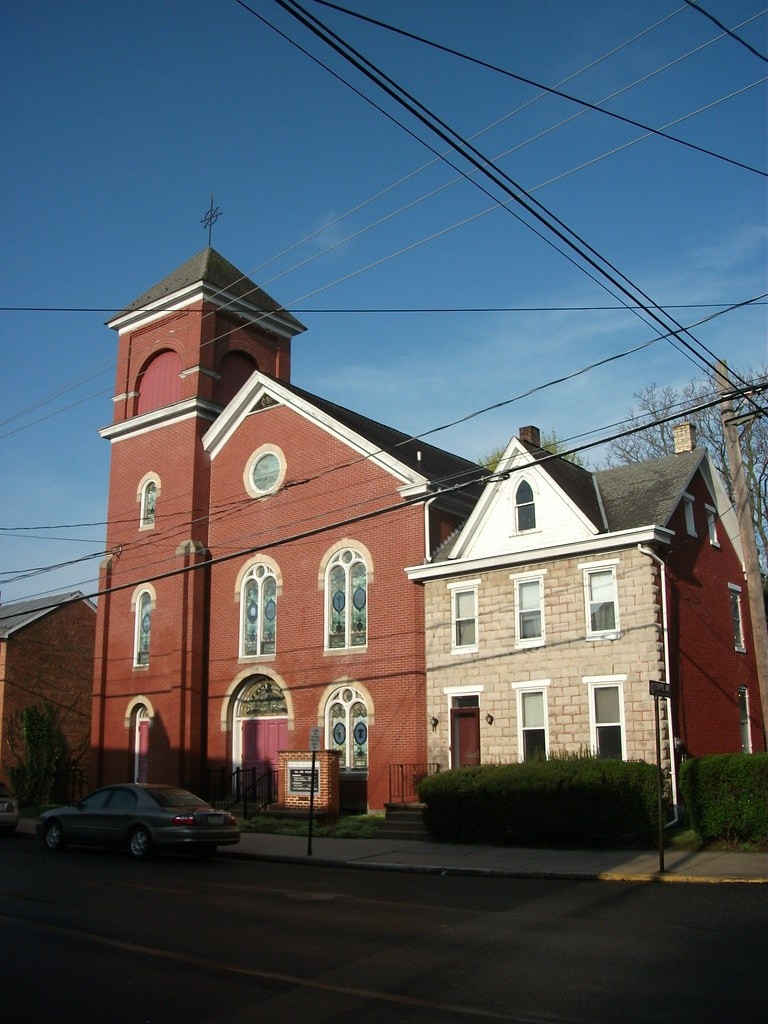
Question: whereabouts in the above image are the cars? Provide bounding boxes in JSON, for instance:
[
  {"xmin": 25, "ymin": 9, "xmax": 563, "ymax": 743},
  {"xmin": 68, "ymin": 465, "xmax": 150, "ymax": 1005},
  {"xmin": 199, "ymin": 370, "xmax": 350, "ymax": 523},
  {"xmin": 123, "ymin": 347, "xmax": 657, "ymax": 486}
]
[
  {"xmin": 35, "ymin": 782, "xmax": 241, "ymax": 862},
  {"xmin": 0, "ymin": 783, "xmax": 20, "ymax": 833}
]
